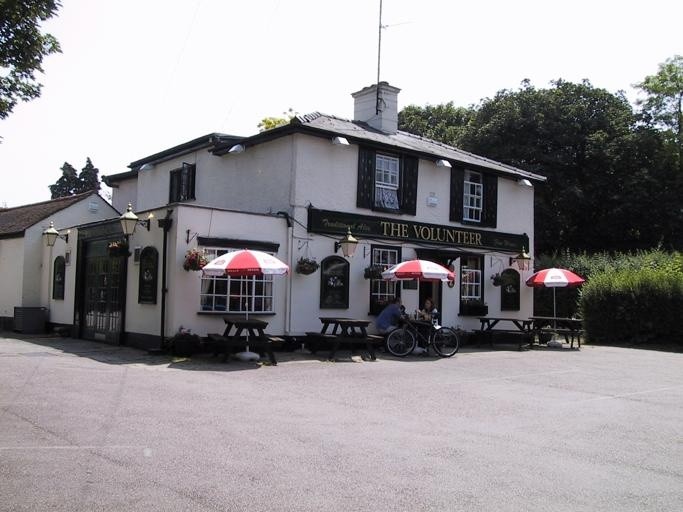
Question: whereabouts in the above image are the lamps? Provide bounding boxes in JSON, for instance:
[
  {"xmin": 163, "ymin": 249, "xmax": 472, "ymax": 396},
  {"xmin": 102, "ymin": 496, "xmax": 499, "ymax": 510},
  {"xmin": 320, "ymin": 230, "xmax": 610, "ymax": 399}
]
[
  {"xmin": 227, "ymin": 143, "xmax": 245, "ymax": 156},
  {"xmin": 434, "ymin": 159, "xmax": 452, "ymax": 169},
  {"xmin": 333, "ymin": 225, "xmax": 358, "ymax": 258},
  {"xmin": 331, "ymin": 136, "xmax": 350, "ymax": 147},
  {"xmin": 41, "ymin": 220, "xmax": 68, "ymax": 247},
  {"xmin": 517, "ymin": 178, "xmax": 532, "ymax": 190},
  {"xmin": 508, "ymin": 244, "xmax": 531, "ymax": 271},
  {"xmin": 118, "ymin": 201, "xmax": 150, "ymax": 237}
]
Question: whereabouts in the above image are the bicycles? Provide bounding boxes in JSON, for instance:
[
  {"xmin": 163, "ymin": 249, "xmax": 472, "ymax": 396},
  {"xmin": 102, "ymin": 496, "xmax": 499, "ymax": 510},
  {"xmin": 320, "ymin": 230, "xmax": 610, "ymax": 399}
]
[{"xmin": 385, "ymin": 305, "xmax": 460, "ymax": 358}]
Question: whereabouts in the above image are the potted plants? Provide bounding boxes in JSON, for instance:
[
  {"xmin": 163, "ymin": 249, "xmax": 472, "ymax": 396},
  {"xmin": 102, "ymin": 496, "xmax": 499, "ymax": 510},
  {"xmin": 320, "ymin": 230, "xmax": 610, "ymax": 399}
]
[
  {"xmin": 363, "ymin": 263, "xmax": 381, "ymax": 281},
  {"xmin": 295, "ymin": 256, "xmax": 318, "ymax": 274}
]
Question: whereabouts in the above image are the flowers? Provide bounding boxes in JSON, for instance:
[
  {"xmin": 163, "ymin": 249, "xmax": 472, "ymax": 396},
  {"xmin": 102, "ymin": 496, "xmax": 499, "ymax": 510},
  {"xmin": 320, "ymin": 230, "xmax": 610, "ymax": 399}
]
[{"xmin": 182, "ymin": 247, "xmax": 207, "ymax": 271}]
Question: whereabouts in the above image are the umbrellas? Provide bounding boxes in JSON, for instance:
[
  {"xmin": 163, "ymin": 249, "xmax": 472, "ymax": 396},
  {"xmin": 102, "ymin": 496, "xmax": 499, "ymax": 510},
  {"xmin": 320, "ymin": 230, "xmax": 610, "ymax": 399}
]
[
  {"xmin": 525, "ymin": 267, "xmax": 585, "ymax": 341},
  {"xmin": 380, "ymin": 258, "xmax": 455, "ymax": 347},
  {"xmin": 202, "ymin": 248, "xmax": 289, "ymax": 352}
]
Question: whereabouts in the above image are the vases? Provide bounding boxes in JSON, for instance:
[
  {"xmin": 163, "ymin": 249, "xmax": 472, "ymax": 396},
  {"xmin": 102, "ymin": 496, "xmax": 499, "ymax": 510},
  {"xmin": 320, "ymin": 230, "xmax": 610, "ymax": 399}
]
[{"xmin": 187, "ymin": 269, "xmax": 203, "ymax": 277}]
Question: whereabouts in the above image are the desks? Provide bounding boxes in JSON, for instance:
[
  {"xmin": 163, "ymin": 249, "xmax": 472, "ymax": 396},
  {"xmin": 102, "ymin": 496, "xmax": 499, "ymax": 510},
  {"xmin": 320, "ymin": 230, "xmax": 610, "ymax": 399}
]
[
  {"xmin": 318, "ymin": 317, "xmax": 372, "ymax": 337},
  {"xmin": 221, "ymin": 318, "xmax": 267, "ymax": 337},
  {"xmin": 474, "ymin": 315, "xmax": 583, "ymax": 331}
]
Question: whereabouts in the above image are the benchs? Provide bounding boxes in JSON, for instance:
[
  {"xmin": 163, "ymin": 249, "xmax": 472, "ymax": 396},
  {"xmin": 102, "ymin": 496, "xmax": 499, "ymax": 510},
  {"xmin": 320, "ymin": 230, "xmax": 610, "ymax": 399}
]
[
  {"xmin": 470, "ymin": 327, "xmax": 586, "ymax": 350},
  {"xmin": 207, "ymin": 332, "xmax": 284, "ymax": 365},
  {"xmin": 304, "ymin": 330, "xmax": 384, "ymax": 360}
]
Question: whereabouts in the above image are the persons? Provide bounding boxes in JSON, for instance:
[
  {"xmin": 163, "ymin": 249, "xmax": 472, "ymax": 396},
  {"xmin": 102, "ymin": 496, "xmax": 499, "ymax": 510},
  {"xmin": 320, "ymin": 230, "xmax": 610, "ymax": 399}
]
[
  {"xmin": 376, "ymin": 297, "xmax": 406, "ymax": 353},
  {"xmin": 414, "ymin": 298, "xmax": 440, "ymax": 353}
]
